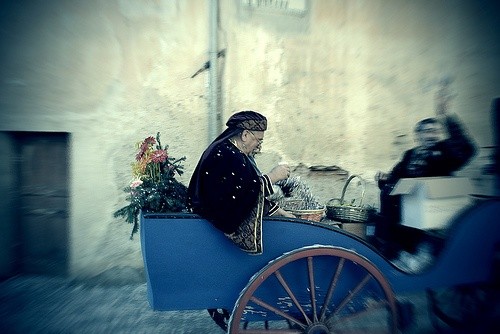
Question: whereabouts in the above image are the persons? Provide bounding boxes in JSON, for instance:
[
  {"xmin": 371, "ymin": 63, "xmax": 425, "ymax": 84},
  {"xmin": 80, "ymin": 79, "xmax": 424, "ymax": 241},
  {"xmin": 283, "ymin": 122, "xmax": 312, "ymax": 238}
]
[
  {"xmin": 187, "ymin": 110, "xmax": 297, "ymax": 256},
  {"xmin": 373, "ymin": 80, "xmax": 479, "ymax": 266}
]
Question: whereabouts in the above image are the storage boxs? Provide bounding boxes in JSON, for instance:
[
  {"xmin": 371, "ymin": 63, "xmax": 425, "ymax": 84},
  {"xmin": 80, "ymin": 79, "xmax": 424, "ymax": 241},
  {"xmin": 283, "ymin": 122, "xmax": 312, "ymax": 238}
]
[
  {"xmin": 320, "ymin": 213, "xmax": 379, "ymax": 245},
  {"xmin": 389, "ymin": 176, "xmax": 477, "ymax": 229}
]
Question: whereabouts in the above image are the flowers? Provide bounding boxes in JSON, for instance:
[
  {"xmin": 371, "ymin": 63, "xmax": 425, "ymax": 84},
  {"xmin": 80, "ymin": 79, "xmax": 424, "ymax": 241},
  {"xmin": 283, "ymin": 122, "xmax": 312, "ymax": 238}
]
[{"xmin": 113, "ymin": 132, "xmax": 187, "ymax": 239}]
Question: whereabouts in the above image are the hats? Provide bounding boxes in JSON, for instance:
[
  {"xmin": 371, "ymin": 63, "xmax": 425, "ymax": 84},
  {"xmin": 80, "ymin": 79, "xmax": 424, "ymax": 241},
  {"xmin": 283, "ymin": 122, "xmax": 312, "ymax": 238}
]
[{"xmin": 226, "ymin": 111, "xmax": 267, "ymax": 131}]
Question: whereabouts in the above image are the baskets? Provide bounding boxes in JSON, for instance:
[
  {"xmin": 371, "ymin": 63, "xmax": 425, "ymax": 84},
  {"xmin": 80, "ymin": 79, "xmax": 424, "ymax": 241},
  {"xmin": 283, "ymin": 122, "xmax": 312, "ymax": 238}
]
[
  {"xmin": 327, "ymin": 175, "xmax": 370, "ymax": 223},
  {"xmin": 282, "ymin": 205, "xmax": 325, "ymax": 222}
]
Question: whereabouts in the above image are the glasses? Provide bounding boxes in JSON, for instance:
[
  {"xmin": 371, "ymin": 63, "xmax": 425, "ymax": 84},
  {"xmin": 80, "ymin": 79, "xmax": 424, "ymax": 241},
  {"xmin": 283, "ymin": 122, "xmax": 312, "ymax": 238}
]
[{"xmin": 247, "ymin": 130, "xmax": 263, "ymax": 144}]
